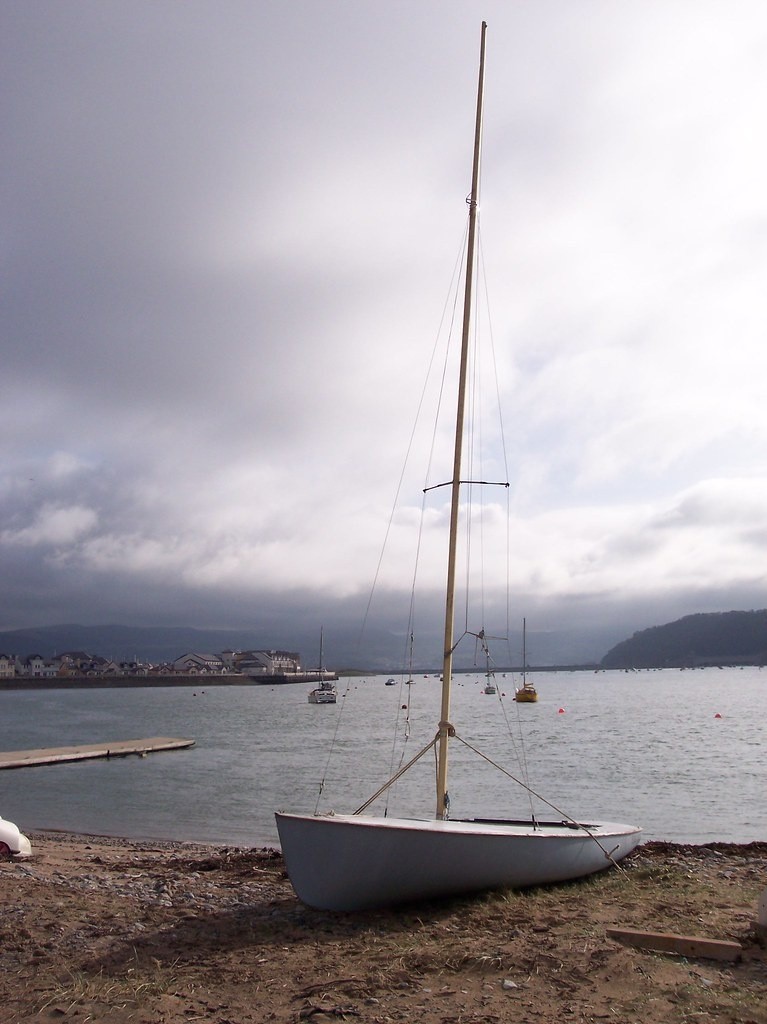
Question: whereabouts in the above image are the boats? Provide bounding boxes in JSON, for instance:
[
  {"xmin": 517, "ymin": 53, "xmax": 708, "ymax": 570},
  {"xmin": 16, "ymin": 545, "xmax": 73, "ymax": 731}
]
[{"xmin": 385, "ymin": 679, "xmax": 396, "ymax": 686}]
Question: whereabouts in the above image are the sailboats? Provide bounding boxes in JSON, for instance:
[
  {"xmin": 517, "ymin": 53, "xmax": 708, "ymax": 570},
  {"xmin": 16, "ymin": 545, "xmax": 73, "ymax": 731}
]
[
  {"xmin": 273, "ymin": 19, "xmax": 642, "ymax": 918},
  {"xmin": 308, "ymin": 624, "xmax": 336, "ymax": 704},
  {"xmin": 514, "ymin": 616, "xmax": 538, "ymax": 703},
  {"xmin": 485, "ymin": 658, "xmax": 495, "ymax": 694}
]
